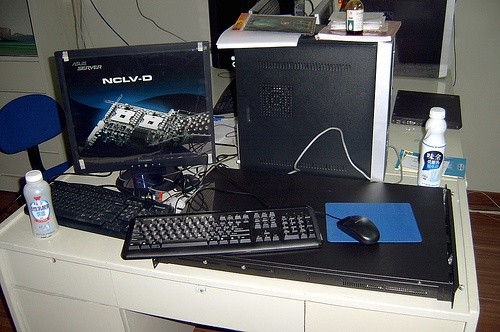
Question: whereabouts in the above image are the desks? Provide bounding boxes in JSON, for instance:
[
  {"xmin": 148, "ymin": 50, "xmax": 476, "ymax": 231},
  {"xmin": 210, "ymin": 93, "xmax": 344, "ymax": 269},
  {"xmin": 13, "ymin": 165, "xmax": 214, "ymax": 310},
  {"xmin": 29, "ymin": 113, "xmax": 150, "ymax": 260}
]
[
  {"xmin": 0, "ymin": 158, "xmax": 480, "ymax": 319},
  {"xmin": 211, "ymin": 66, "xmax": 465, "ymax": 178}
]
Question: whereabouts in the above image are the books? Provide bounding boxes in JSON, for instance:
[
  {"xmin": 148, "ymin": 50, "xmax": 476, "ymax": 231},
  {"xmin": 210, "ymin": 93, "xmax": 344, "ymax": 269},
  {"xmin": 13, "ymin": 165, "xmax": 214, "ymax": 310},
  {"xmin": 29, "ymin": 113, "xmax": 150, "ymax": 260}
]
[{"xmin": 314, "ymin": 12, "xmax": 402, "ymax": 41}]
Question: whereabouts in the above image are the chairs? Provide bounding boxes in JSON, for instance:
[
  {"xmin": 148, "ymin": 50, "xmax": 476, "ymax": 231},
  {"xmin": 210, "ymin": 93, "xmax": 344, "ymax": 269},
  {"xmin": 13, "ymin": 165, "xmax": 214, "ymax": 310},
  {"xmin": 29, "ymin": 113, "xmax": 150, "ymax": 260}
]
[{"xmin": 0, "ymin": 94, "xmax": 70, "ymax": 185}]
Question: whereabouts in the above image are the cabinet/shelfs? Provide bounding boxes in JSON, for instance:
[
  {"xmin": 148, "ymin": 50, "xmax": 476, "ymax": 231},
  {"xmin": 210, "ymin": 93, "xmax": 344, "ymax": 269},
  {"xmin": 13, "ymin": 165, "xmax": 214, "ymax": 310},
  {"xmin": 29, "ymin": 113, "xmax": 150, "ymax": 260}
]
[
  {"xmin": 0, "ymin": 249, "xmax": 465, "ymax": 331},
  {"xmin": 0, "ymin": 0, "xmax": 76, "ymax": 194}
]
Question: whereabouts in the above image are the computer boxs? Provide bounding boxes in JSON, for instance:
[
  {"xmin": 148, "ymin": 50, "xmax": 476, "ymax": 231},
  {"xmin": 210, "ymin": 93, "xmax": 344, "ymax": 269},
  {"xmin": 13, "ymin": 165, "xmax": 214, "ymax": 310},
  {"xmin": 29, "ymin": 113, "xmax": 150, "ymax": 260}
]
[{"xmin": 231, "ymin": 32, "xmax": 395, "ymax": 184}]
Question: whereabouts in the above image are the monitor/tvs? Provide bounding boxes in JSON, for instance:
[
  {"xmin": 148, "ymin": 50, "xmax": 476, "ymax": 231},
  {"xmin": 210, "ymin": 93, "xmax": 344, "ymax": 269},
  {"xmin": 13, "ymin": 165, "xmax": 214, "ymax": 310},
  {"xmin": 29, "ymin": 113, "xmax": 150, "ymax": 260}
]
[
  {"xmin": 54, "ymin": 40, "xmax": 216, "ymax": 194},
  {"xmin": 204, "ymin": 1, "xmax": 455, "ymax": 78}
]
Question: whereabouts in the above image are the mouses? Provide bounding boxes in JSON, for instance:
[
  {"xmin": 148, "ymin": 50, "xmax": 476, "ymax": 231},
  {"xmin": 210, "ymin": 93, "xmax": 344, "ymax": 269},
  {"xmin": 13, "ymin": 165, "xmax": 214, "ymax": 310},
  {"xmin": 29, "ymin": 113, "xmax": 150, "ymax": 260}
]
[{"xmin": 336, "ymin": 215, "xmax": 381, "ymax": 245}]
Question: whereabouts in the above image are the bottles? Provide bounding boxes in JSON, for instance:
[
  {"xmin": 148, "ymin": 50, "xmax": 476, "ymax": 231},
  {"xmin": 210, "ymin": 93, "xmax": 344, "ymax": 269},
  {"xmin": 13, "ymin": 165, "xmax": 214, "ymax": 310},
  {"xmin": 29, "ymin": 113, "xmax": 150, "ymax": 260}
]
[
  {"xmin": 23, "ymin": 170, "xmax": 59, "ymax": 238},
  {"xmin": 418, "ymin": 107, "xmax": 447, "ymax": 188},
  {"xmin": 346, "ymin": 0, "xmax": 364, "ymax": 35}
]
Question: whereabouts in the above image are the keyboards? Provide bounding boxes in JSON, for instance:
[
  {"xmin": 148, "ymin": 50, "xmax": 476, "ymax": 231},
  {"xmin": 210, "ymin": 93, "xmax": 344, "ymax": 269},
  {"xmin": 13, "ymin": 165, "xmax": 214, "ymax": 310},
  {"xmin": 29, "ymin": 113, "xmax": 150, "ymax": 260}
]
[
  {"xmin": 120, "ymin": 205, "xmax": 324, "ymax": 261},
  {"xmin": 24, "ymin": 181, "xmax": 177, "ymax": 241}
]
